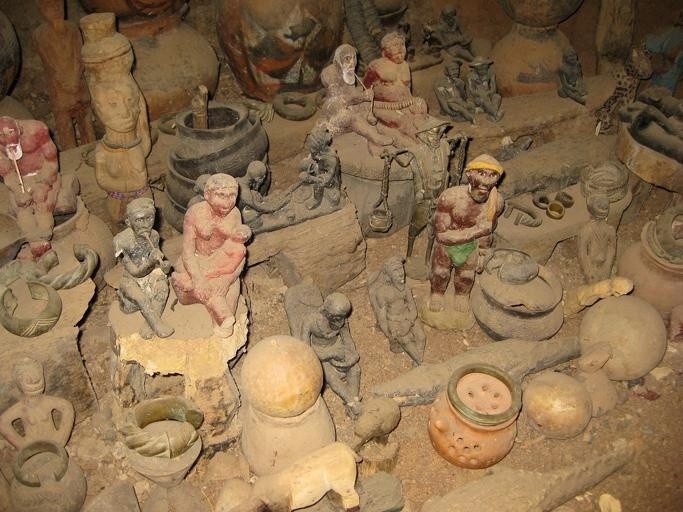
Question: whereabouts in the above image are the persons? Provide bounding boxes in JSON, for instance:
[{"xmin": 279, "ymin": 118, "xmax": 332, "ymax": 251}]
[{"xmin": 0, "ymin": 1, "xmax": 619, "ymax": 450}]
